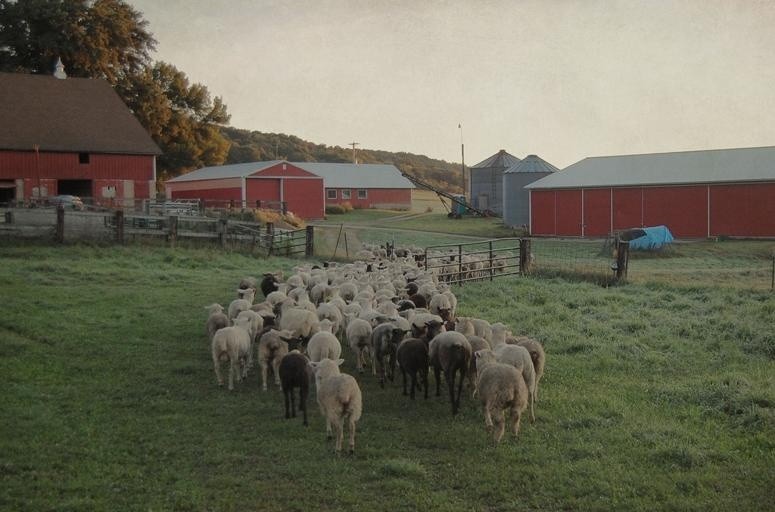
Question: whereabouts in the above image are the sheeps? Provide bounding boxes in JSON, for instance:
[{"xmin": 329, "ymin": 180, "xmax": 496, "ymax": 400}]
[{"xmin": 204, "ymin": 242, "xmax": 545, "ymax": 456}]
